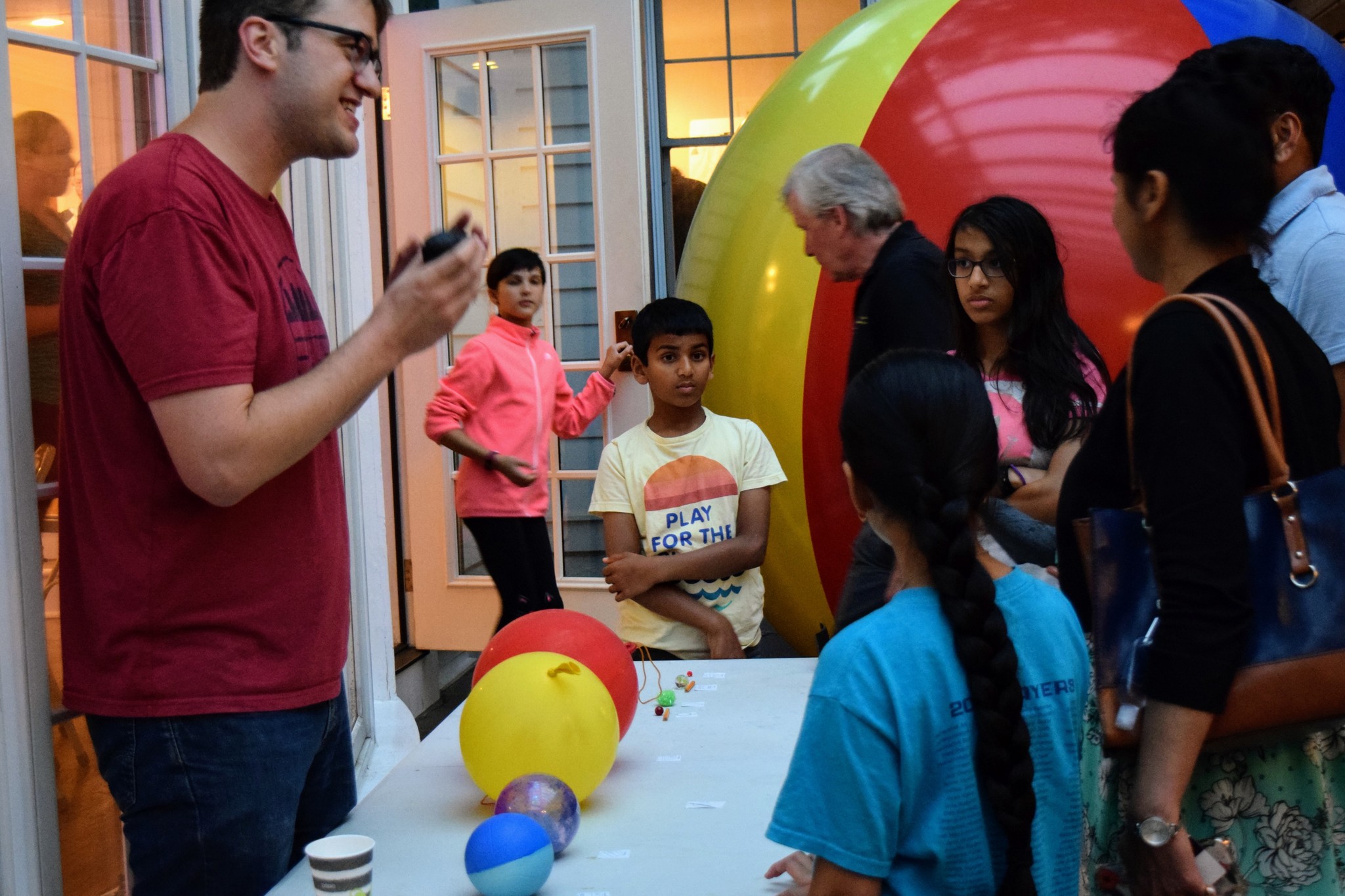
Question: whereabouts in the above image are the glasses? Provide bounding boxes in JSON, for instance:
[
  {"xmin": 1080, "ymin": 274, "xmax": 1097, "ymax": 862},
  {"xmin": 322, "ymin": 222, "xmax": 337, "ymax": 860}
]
[
  {"xmin": 265, "ymin": 15, "xmax": 382, "ymax": 79},
  {"xmin": 947, "ymin": 257, "xmax": 1007, "ymax": 278}
]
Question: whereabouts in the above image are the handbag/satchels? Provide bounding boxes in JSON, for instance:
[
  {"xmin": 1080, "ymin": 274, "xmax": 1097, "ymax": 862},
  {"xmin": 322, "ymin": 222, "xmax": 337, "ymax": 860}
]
[{"xmin": 1070, "ymin": 293, "xmax": 1345, "ymax": 752}]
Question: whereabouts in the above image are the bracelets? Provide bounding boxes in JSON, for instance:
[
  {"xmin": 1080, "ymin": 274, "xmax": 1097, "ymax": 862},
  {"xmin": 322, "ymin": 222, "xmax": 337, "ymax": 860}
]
[{"xmin": 485, "ymin": 450, "xmax": 500, "ymax": 470}]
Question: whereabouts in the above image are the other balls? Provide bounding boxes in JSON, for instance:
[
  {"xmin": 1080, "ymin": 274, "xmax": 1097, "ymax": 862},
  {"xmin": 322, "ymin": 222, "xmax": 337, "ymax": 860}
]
[
  {"xmin": 494, "ymin": 773, "xmax": 578, "ymax": 856},
  {"xmin": 464, "ymin": 810, "xmax": 553, "ymax": 896}
]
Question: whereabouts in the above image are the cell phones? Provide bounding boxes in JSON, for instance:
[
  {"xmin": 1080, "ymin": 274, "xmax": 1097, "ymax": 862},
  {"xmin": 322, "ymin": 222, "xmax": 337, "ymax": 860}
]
[{"xmin": 1188, "ymin": 836, "xmax": 1250, "ymax": 896}]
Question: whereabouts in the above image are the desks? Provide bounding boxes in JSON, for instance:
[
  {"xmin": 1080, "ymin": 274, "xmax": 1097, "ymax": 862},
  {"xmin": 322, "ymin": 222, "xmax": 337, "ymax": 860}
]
[{"xmin": 265, "ymin": 657, "xmax": 818, "ymax": 896}]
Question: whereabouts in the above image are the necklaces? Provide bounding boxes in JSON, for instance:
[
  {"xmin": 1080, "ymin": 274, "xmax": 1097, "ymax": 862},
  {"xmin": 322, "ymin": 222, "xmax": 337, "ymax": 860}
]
[{"xmin": 765, "ymin": 346, "xmax": 1090, "ymax": 896}]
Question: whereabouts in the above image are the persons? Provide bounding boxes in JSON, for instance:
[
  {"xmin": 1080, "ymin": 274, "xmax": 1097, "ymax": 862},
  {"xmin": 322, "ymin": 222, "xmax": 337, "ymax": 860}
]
[
  {"xmin": 53, "ymin": 0, "xmax": 487, "ymax": 896},
  {"xmin": 587, "ymin": 298, "xmax": 797, "ymax": 659},
  {"xmin": 1056, "ymin": 66, "xmax": 1343, "ymax": 896},
  {"xmin": 779, "ymin": 144, "xmax": 986, "ymax": 628},
  {"xmin": 1170, "ymin": 38, "xmax": 1345, "ymax": 386},
  {"xmin": 942, "ymin": 198, "xmax": 1109, "ymax": 527},
  {"xmin": 10, "ymin": 110, "xmax": 82, "ymax": 483},
  {"xmin": 426, "ymin": 250, "xmax": 635, "ymax": 633}
]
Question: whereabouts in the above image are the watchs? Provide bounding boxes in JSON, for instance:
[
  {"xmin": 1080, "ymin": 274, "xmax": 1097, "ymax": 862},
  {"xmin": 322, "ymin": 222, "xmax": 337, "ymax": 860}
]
[{"xmin": 1130, "ymin": 817, "xmax": 1181, "ymax": 849}]
